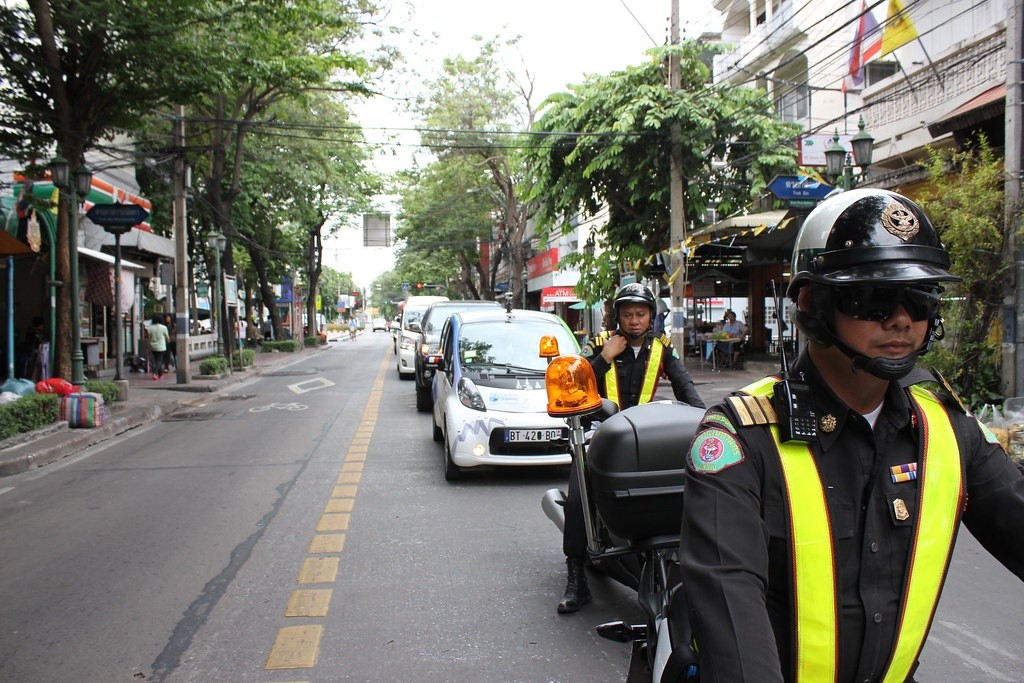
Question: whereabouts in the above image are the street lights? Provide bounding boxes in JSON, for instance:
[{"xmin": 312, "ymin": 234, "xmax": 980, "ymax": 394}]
[
  {"xmin": 207, "ymin": 223, "xmax": 227, "ymax": 357},
  {"xmin": 50, "ymin": 154, "xmax": 94, "ymax": 394},
  {"xmin": 824, "ymin": 114, "xmax": 875, "ymax": 191},
  {"xmin": 583, "ymin": 238, "xmax": 595, "ymax": 335},
  {"xmin": 521, "ymin": 267, "xmax": 529, "ymax": 309}
]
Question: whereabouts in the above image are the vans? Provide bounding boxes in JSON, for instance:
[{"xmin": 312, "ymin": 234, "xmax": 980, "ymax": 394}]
[
  {"xmin": 391, "ymin": 297, "xmax": 452, "ymax": 377},
  {"xmin": 372, "ymin": 318, "xmax": 387, "ymax": 332}
]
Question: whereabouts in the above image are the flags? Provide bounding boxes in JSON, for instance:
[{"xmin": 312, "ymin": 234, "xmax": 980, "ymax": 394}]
[
  {"xmin": 879, "ymin": 0, "xmax": 919, "ymax": 61},
  {"xmin": 848, "ymin": 0, "xmax": 884, "ymax": 86}
]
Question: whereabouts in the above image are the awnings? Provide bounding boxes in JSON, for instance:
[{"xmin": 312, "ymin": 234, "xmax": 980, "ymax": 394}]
[
  {"xmin": 567, "ymin": 300, "xmax": 605, "ymax": 310},
  {"xmin": 615, "ymin": 208, "xmax": 791, "ymax": 274}
]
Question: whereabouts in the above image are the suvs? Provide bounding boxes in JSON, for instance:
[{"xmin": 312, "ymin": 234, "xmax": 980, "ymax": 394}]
[{"xmin": 408, "ymin": 301, "xmax": 504, "ymax": 411}]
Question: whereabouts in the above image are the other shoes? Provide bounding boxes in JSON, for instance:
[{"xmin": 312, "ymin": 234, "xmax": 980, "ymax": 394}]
[
  {"xmin": 162, "ymin": 369, "xmax": 168, "ymax": 373},
  {"xmin": 158, "ymin": 375, "xmax": 165, "ymax": 379},
  {"xmin": 152, "ymin": 373, "xmax": 157, "ymax": 380}
]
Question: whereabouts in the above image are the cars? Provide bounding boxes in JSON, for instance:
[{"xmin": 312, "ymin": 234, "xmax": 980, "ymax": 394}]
[
  {"xmin": 427, "ymin": 307, "xmax": 602, "ymax": 481},
  {"xmin": 393, "ymin": 315, "xmax": 401, "ymax": 353}
]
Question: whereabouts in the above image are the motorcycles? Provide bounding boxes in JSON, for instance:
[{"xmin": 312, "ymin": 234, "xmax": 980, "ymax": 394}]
[{"xmin": 539, "ymin": 337, "xmax": 707, "ymax": 683}]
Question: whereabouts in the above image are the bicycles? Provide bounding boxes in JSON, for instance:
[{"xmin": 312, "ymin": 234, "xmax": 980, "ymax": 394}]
[{"xmin": 350, "ymin": 329, "xmax": 355, "ymax": 341}]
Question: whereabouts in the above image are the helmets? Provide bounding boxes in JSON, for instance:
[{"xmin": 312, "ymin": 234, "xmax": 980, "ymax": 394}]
[
  {"xmin": 615, "ymin": 283, "xmax": 658, "ymax": 321},
  {"xmin": 785, "ymin": 187, "xmax": 964, "ymax": 296}
]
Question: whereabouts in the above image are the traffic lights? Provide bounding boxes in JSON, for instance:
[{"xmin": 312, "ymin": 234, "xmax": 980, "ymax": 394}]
[{"xmin": 411, "ymin": 283, "xmax": 424, "ymax": 288}]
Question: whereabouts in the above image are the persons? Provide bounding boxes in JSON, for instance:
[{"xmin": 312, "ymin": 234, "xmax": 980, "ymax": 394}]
[
  {"xmin": 145, "ymin": 314, "xmax": 176, "ymax": 379},
  {"xmin": 347, "ymin": 316, "xmax": 356, "ymax": 337},
  {"xmin": 557, "ymin": 282, "xmax": 706, "ymax": 613},
  {"xmin": 189, "ymin": 322, "xmax": 210, "ymax": 334},
  {"xmin": 722, "ymin": 311, "xmax": 748, "ymax": 368},
  {"xmin": 233, "ymin": 316, "xmax": 272, "ymax": 349},
  {"xmin": 678, "ymin": 186, "xmax": 1023, "ymax": 683}
]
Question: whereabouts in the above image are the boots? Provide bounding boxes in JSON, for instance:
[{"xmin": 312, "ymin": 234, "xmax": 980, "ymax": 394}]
[{"xmin": 557, "ymin": 556, "xmax": 593, "ymax": 613}]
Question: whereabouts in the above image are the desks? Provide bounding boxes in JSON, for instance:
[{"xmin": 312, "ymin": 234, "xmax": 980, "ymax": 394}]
[
  {"xmin": 80, "ymin": 337, "xmax": 101, "ymax": 378},
  {"xmin": 698, "ymin": 337, "xmax": 741, "ymax": 372}
]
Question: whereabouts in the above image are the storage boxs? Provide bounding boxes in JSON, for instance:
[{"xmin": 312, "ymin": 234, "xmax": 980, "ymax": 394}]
[{"xmin": 586, "ymin": 400, "xmax": 708, "ymax": 539}]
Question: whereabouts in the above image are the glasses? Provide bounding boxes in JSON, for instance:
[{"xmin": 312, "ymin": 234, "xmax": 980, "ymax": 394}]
[{"xmin": 830, "ymin": 290, "xmax": 944, "ymax": 323}]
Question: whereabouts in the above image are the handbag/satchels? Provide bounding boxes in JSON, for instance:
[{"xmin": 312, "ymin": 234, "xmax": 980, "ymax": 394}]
[
  {"xmin": 37, "ymin": 378, "xmax": 79, "ymax": 396},
  {"xmin": 61, "ymin": 392, "xmax": 105, "ymax": 427}
]
